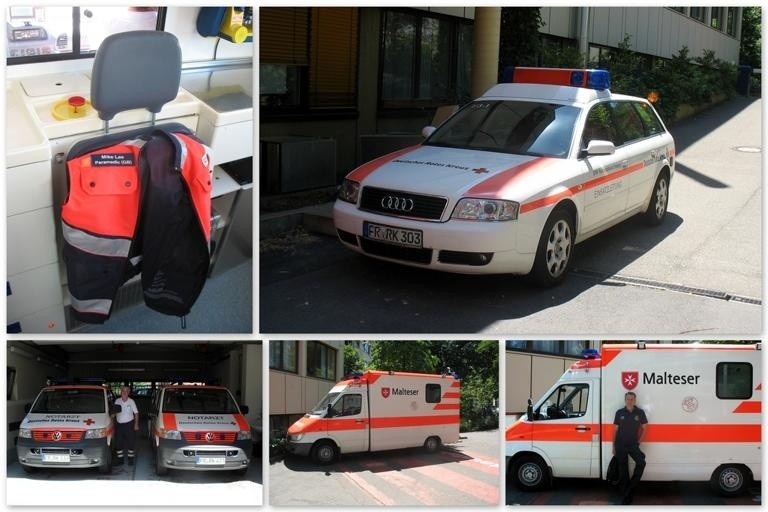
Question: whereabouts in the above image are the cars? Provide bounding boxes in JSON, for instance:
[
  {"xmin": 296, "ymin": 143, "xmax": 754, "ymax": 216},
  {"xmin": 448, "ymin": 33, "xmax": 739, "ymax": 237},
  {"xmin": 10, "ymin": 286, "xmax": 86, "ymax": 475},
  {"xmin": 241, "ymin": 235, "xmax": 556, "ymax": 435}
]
[{"xmin": 333, "ymin": 64, "xmax": 676, "ymax": 287}]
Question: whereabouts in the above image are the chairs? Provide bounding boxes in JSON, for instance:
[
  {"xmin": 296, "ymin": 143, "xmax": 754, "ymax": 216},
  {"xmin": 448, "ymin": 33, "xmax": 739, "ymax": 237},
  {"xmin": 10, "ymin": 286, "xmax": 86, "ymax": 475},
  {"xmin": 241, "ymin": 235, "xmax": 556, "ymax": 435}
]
[
  {"xmin": 57, "ymin": 30, "xmax": 213, "ymax": 329},
  {"xmin": 341, "ymin": 409, "xmax": 360, "ymax": 416}
]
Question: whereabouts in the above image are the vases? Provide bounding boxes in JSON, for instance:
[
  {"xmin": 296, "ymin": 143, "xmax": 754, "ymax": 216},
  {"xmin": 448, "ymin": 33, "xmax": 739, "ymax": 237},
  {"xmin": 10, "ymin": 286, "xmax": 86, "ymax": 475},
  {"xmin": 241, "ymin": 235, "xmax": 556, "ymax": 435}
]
[
  {"xmin": 303, "ymin": 249, "xmax": 320, "ymax": 271},
  {"xmin": 268, "ymin": 256, "xmax": 309, "ymax": 288}
]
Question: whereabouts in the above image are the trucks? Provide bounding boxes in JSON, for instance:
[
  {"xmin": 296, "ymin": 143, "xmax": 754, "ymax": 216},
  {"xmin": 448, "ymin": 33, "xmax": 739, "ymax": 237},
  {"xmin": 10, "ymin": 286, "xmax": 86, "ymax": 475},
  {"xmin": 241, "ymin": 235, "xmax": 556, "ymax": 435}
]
[
  {"xmin": 285, "ymin": 368, "xmax": 461, "ymax": 463},
  {"xmin": 506, "ymin": 340, "xmax": 762, "ymax": 495}
]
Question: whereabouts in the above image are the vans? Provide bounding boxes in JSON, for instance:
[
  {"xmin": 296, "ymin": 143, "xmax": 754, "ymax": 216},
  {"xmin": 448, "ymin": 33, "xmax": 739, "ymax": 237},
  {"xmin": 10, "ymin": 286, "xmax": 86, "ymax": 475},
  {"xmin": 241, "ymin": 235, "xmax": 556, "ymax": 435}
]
[
  {"xmin": 16, "ymin": 379, "xmax": 117, "ymax": 475},
  {"xmin": 146, "ymin": 385, "xmax": 253, "ymax": 477}
]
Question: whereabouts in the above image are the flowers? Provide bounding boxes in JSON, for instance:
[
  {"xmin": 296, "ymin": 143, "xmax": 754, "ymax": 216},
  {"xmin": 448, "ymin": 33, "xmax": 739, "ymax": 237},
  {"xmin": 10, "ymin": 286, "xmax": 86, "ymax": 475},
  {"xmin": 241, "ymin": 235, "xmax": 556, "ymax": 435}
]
[
  {"xmin": 298, "ymin": 234, "xmax": 323, "ymax": 252},
  {"xmin": 260, "ymin": 230, "xmax": 302, "ymax": 266}
]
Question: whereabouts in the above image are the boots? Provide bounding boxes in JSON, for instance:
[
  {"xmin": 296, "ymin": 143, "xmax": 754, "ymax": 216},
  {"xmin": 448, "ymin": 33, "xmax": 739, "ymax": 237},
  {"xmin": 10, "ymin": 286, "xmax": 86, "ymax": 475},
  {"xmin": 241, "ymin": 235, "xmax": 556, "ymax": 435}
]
[
  {"xmin": 113, "ymin": 450, "xmax": 124, "ymax": 465},
  {"xmin": 128, "ymin": 450, "xmax": 135, "ymax": 465}
]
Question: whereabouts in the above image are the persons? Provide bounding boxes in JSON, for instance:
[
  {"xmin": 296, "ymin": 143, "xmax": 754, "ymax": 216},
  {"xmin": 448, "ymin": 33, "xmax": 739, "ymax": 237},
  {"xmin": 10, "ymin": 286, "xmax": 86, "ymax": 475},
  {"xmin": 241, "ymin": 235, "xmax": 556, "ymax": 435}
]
[
  {"xmin": 611, "ymin": 391, "xmax": 648, "ymax": 505},
  {"xmin": 111, "ymin": 385, "xmax": 139, "ymax": 467}
]
